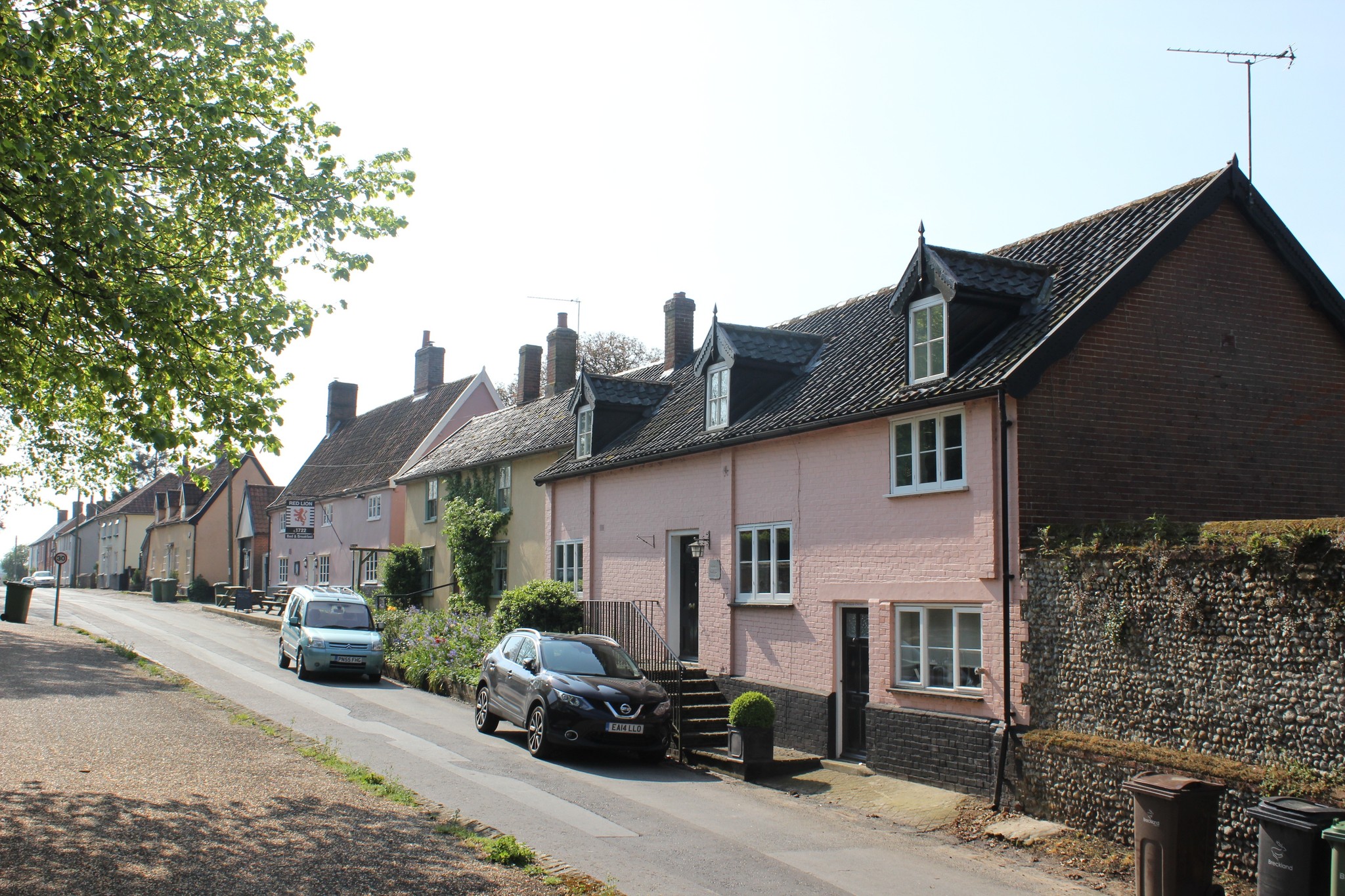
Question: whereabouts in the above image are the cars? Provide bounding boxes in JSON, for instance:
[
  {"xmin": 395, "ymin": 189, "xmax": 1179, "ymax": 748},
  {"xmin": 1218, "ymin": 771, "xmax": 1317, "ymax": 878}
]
[
  {"xmin": 21, "ymin": 577, "xmax": 31, "ymax": 585},
  {"xmin": 30, "ymin": 570, "xmax": 56, "ymax": 587}
]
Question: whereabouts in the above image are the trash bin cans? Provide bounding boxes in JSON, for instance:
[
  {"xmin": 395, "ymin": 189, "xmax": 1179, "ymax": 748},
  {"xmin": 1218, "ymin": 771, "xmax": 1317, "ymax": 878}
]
[
  {"xmin": 1121, "ymin": 770, "xmax": 1225, "ymax": 896},
  {"xmin": 213, "ymin": 582, "xmax": 230, "ymax": 606},
  {"xmin": 1322, "ymin": 817, "xmax": 1345, "ymax": 896},
  {"xmin": 149, "ymin": 578, "xmax": 163, "ymax": 601},
  {"xmin": 159, "ymin": 578, "xmax": 178, "ymax": 602},
  {"xmin": 1244, "ymin": 796, "xmax": 1344, "ymax": 896},
  {"xmin": 0, "ymin": 580, "xmax": 37, "ymax": 623}
]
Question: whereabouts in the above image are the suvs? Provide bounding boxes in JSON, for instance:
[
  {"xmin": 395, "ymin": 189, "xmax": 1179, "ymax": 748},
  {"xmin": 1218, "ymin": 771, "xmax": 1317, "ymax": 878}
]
[
  {"xmin": 471, "ymin": 626, "xmax": 675, "ymax": 766},
  {"xmin": 277, "ymin": 583, "xmax": 384, "ymax": 683}
]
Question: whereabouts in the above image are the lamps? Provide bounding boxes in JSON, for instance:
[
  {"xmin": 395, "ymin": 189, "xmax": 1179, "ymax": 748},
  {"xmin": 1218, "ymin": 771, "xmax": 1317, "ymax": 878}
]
[
  {"xmin": 354, "ymin": 493, "xmax": 365, "ymax": 500},
  {"xmin": 313, "ymin": 557, "xmax": 318, "ymax": 568},
  {"xmin": 302, "ymin": 558, "xmax": 307, "ymax": 569},
  {"xmin": 688, "ymin": 535, "xmax": 711, "ymax": 557},
  {"xmin": 241, "ymin": 548, "xmax": 250, "ymax": 557}
]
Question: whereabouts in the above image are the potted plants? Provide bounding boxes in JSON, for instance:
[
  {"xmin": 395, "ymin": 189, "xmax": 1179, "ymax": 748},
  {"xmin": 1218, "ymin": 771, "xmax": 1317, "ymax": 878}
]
[{"xmin": 726, "ymin": 692, "xmax": 778, "ymax": 765}]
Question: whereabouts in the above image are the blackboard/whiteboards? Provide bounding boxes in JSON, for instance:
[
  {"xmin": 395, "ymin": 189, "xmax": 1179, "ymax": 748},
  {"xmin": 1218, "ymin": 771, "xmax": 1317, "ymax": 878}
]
[{"xmin": 235, "ymin": 589, "xmax": 252, "ymax": 609}]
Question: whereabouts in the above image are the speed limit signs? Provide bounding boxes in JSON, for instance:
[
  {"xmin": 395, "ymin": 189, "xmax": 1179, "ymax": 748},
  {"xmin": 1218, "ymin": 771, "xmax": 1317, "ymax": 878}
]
[{"xmin": 54, "ymin": 552, "xmax": 67, "ymax": 564}]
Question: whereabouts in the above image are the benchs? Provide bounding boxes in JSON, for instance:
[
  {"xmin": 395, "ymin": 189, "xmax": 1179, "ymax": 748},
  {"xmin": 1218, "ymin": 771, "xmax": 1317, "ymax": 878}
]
[{"xmin": 216, "ymin": 584, "xmax": 292, "ymax": 615}]
